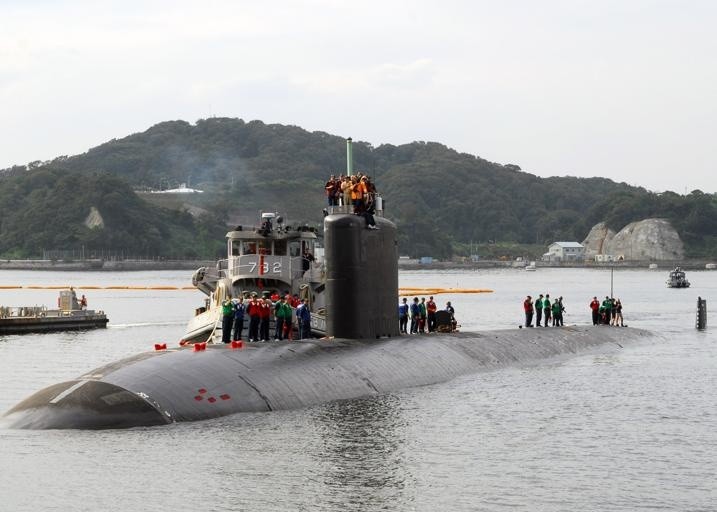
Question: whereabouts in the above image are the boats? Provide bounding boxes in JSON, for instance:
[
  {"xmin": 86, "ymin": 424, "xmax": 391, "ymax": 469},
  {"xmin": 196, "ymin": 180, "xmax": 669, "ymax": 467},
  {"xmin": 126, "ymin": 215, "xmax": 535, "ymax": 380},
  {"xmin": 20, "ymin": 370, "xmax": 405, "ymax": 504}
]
[
  {"xmin": 173, "ymin": 208, "xmax": 324, "ymax": 346},
  {"xmin": 0, "ymin": 288, "xmax": 114, "ymax": 339},
  {"xmin": 524, "ymin": 265, "xmax": 536, "ymax": 271},
  {"xmin": 664, "ymin": 265, "xmax": 691, "ymax": 288}
]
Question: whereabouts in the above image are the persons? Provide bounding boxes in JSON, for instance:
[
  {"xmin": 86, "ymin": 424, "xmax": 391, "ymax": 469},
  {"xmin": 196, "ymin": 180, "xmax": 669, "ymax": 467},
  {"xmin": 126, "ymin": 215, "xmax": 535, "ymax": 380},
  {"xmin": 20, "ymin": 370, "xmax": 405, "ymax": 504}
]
[
  {"xmin": 524, "ymin": 294, "xmax": 566, "ymax": 327},
  {"xmin": 324, "ymin": 169, "xmax": 379, "ymax": 231},
  {"xmin": 590, "ymin": 296, "xmax": 624, "ymax": 327},
  {"xmin": 222, "ymin": 291, "xmax": 311, "ymax": 344},
  {"xmin": 446, "ymin": 302, "xmax": 455, "ymax": 321},
  {"xmin": 399, "ymin": 296, "xmax": 437, "ymax": 334},
  {"xmin": 69, "ymin": 287, "xmax": 87, "ymax": 311},
  {"xmin": 239, "ymin": 217, "xmax": 315, "ymax": 275}
]
